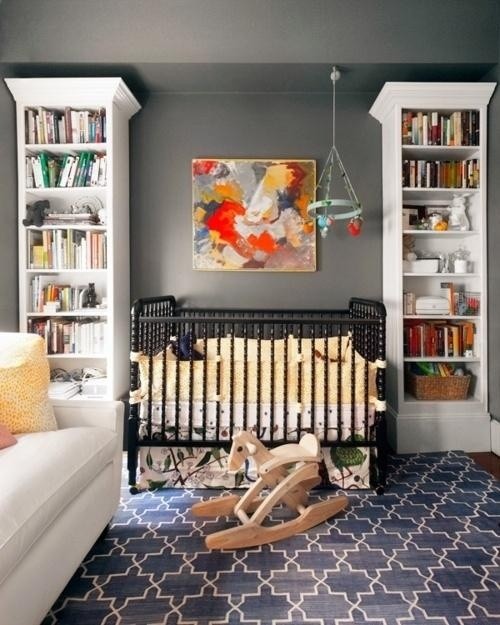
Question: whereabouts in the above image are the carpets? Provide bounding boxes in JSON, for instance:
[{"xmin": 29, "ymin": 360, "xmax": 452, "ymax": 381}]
[{"xmin": 41, "ymin": 448, "xmax": 500, "ymax": 625}]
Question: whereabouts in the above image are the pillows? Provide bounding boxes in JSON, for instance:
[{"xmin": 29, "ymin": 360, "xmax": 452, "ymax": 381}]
[{"xmin": 0, "ymin": 331, "xmax": 58, "ymax": 449}]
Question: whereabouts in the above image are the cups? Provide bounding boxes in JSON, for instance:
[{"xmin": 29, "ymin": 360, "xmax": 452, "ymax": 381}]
[{"xmin": 454, "ymin": 260, "xmax": 467, "ymax": 274}]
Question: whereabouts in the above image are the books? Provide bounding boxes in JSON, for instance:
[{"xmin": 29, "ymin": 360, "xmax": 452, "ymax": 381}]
[
  {"xmin": 21, "ymin": 110, "xmax": 113, "ymax": 355},
  {"xmin": 400, "ymin": 108, "xmax": 480, "ymax": 187},
  {"xmin": 404, "ymin": 318, "xmax": 477, "ymax": 358}
]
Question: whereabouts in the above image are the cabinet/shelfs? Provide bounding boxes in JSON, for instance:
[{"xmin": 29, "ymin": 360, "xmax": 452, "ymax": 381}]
[
  {"xmin": 3, "ymin": 78, "xmax": 143, "ymax": 400},
  {"xmin": 369, "ymin": 81, "xmax": 498, "ymax": 415}
]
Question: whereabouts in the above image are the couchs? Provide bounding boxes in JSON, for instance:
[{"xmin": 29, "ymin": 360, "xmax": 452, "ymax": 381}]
[{"xmin": 0, "ymin": 400, "xmax": 125, "ymax": 625}]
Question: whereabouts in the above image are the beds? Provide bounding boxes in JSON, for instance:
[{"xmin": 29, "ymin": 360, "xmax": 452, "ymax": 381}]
[{"xmin": 128, "ymin": 296, "xmax": 388, "ymax": 496}]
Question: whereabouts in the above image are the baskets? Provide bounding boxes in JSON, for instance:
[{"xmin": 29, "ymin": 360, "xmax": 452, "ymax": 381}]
[{"xmin": 406, "ymin": 373, "xmax": 470, "ymax": 402}]
